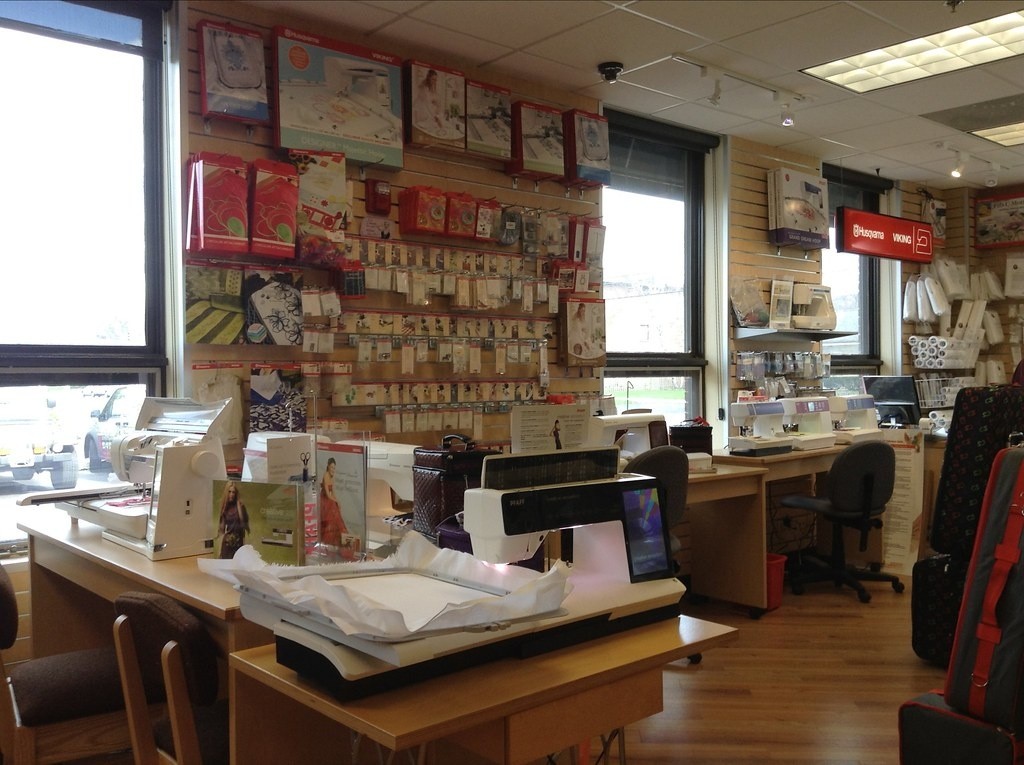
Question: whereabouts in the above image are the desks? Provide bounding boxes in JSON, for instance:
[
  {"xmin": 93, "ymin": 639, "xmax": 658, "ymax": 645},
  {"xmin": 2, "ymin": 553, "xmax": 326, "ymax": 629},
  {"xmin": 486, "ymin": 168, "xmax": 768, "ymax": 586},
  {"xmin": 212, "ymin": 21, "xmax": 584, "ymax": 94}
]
[
  {"xmin": 712, "ymin": 442, "xmax": 885, "ymax": 574},
  {"xmin": 19, "ymin": 518, "xmax": 741, "ymax": 765},
  {"xmin": 668, "ymin": 463, "xmax": 771, "ymax": 620},
  {"xmin": 917, "ymin": 438, "xmax": 949, "ymax": 562}
]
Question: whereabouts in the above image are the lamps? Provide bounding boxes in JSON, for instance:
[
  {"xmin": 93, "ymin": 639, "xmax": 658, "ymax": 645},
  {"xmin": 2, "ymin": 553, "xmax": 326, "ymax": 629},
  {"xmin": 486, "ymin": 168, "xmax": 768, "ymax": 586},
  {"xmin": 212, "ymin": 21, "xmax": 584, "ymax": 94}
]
[
  {"xmin": 984, "ymin": 160, "xmax": 1000, "ymax": 187},
  {"xmin": 701, "ymin": 67, "xmax": 726, "ymax": 108},
  {"xmin": 952, "ymin": 152, "xmax": 968, "ymax": 177},
  {"xmin": 774, "ymin": 90, "xmax": 795, "ymax": 128}
]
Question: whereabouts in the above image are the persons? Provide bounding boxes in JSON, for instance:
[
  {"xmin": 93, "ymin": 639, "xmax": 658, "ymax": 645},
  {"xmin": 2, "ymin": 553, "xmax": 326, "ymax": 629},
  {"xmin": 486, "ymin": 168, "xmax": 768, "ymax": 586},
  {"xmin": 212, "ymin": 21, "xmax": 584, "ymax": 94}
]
[
  {"xmin": 318, "ymin": 457, "xmax": 352, "ymax": 547},
  {"xmin": 413, "ymin": 69, "xmax": 443, "ymax": 129},
  {"xmin": 549, "ymin": 420, "xmax": 562, "ymax": 449},
  {"xmin": 219, "ymin": 480, "xmax": 250, "ymax": 559}
]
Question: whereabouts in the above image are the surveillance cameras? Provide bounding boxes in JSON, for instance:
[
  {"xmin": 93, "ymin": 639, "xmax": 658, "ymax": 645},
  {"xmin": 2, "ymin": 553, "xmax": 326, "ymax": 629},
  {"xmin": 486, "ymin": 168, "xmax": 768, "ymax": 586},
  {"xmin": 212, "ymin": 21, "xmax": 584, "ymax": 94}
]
[{"xmin": 597, "ymin": 62, "xmax": 623, "ymax": 84}]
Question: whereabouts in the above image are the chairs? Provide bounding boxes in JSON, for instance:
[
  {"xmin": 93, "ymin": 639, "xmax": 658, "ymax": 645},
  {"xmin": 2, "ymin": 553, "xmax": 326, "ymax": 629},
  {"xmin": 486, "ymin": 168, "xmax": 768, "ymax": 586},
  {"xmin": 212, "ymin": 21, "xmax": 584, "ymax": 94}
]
[
  {"xmin": 111, "ymin": 590, "xmax": 230, "ymax": 765},
  {"xmin": 780, "ymin": 439, "xmax": 905, "ymax": 603},
  {"xmin": 621, "ymin": 445, "xmax": 703, "ymax": 664},
  {"xmin": 0, "ymin": 562, "xmax": 165, "ymax": 765}
]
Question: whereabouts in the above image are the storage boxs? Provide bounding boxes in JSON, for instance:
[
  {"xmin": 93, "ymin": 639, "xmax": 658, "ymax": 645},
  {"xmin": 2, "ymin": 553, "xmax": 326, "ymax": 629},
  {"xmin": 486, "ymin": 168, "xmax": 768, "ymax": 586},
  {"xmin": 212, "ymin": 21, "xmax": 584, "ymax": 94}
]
[
  {"xmin": 767, "ymin": 167, "xmax": 830, "ymax": 251},
  {"xmin": 185, "ymin": 20, "xmax": 612, "ymax": 266}
]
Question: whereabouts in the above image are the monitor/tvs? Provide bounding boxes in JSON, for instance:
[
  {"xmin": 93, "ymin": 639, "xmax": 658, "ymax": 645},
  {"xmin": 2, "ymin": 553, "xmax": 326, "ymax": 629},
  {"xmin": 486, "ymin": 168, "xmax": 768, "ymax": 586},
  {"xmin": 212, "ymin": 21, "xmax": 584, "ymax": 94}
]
[{"xmin": 863, "ymin": 375, "xmax": 922, "ymax": 429}]
